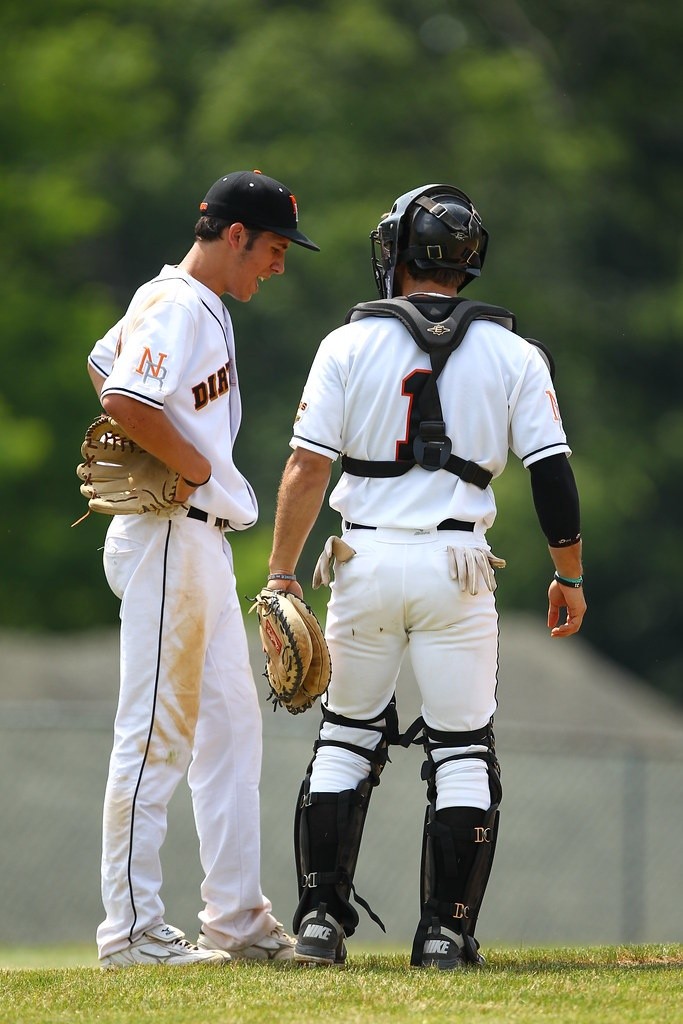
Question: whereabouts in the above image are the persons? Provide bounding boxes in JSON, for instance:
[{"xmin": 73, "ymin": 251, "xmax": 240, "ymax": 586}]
[
  {"xmin": 264, "ymin": 184, "xmax": 584, "ymax": 969},
  {"xmin": 87, "ymin": 169, "xmax": 296, "ymax": 965}
]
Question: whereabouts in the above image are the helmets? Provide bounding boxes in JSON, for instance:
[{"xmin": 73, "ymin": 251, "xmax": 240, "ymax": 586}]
[{"xmin": 369, "ymin": 183, "xmax": 489, "ymax": 300}]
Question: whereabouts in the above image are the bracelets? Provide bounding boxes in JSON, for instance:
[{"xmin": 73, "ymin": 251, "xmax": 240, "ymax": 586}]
[
  {"xmin": 267, "ymin": 575, "xmax": 296, "ymax": 580},
  {"xmin": 181, "ymin": 472, "xmax": 212, "ymax": 488},
  {"xmin": 554, "ymin": 571, "xmax": 583, "ymax": 588}
]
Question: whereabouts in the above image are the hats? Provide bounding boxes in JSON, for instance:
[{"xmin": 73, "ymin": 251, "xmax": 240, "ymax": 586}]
[{"xmin": 201, "ymin": 170, "xmax": 322, "ymax": 253}]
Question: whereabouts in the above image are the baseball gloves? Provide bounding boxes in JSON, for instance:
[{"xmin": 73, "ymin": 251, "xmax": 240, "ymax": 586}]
[
  {"xmin": 246, "ymin": 587, "xmax": 334, "ymax": 715},
  {"xmin": 77, "ymin": 419, "xmax": 191, "ymax": 520}
]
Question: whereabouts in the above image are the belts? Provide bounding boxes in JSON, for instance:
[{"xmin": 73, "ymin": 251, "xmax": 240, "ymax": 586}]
[
  {"xmin": 186, "ymin": 505, "xmax": 229, "ymax": 528},
  {"xmin": 344, "ymin": 517, "xmax": 477, "ymax": 534}
]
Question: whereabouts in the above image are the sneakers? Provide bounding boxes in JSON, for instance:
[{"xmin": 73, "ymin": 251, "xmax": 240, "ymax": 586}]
[
  {"xmin": 295, "ymin": 906, "xmax": 348, "ymax": 969},
  {"xmin": 196, "ymin": 923, "xmax": 298, "ymax": 961},
  {"xmin": 421, "ymin": 926, "xmax": 488, "ymax": 970},
  {"xmin": 99, "ymin": 922, "xmax": 232, "ymax": 966}
]
